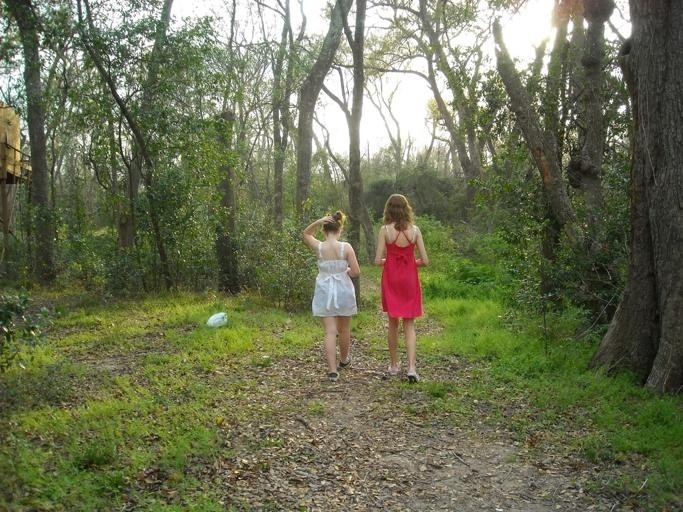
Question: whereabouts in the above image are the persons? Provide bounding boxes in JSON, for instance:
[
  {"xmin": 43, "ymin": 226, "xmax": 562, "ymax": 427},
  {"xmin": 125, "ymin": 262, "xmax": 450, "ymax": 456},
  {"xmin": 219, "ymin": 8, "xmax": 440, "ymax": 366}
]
[
  {"xmin": 375, "ymin": 193, "xmax": 429, "ymax": 383},
  {"xmin": 303, "ymin": 210, "xmax": 361, "ymax": 381}
]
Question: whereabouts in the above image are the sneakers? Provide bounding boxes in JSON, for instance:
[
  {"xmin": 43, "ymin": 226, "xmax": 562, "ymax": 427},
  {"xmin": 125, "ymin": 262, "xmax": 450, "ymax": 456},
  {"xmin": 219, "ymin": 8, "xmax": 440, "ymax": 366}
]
[
  {"xmin": 338, "ymin": 354, "xmax": 351, "ymax": 368},
  {"xmin": 328, "ymin": 372, "xmax": 340, "ymax": 382},
  {"xmin": 407, "ymin": 371, "xmax": 419, "ymax": 383},
  {"xmin": 388, "ymin": 365, "xmax": 399, "ymax": 376}
]
